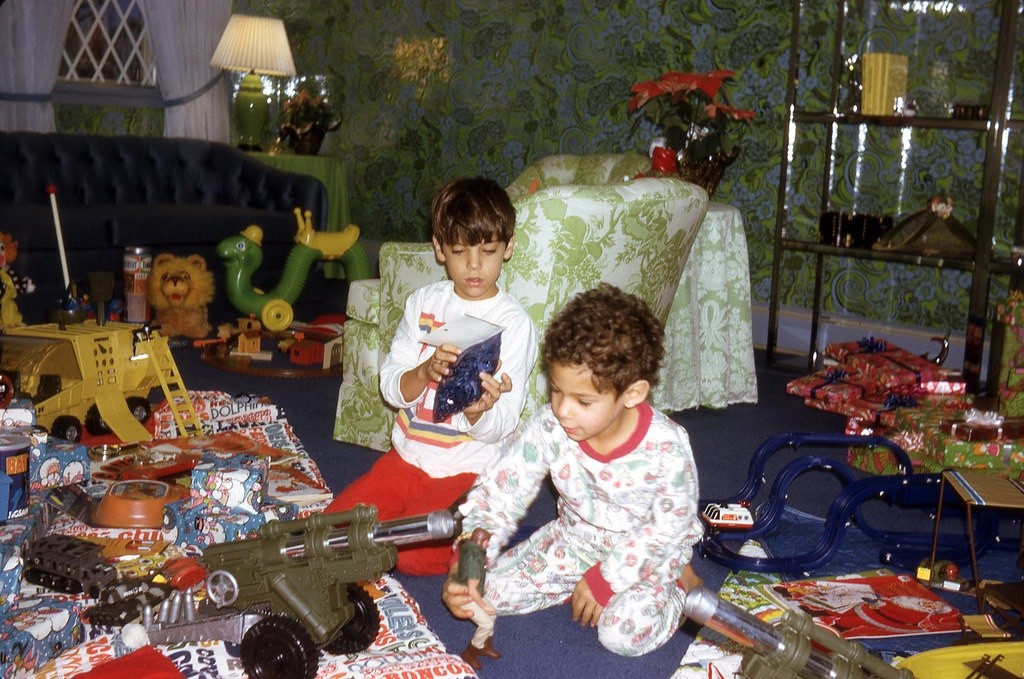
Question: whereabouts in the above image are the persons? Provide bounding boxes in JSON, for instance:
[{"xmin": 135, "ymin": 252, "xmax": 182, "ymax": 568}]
[
  {"xmin": 320, "ymin": 177, "xmax": 539, "ymax": 576},
  {"xmin": 458, "ymin": 528, "xmax": 501, "ymax": 669},
  {"xmin": 441, "ymin": 281, "xmax": 703, "ymax": 657}
]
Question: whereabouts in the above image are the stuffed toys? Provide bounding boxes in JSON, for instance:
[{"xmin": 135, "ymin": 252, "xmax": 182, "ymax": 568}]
[
  {"xmin": 0, "ymin": 233, "xmax": 35, "ymax": 330},
  {"xmin": 148, "ymin": 253, "xmax": 213, "ymax": 338}
]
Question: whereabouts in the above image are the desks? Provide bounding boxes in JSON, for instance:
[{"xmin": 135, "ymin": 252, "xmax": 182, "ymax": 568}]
[
  {"xmin": 648, "ymin": 202, "xmax": 759, "ymax": 415},
  {"xmin": 247, "ymin": 150, "xmax": 350, "ymax": 280},
  {"xmin": 927, "ymin": 468, "xmax": 1022, "ymax": 614}
]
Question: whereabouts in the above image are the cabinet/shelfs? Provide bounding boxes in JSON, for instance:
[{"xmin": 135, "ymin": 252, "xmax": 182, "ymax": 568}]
[{"xmin": 763, "ymin": 0, "xmax": 1024, "ymax": 394}]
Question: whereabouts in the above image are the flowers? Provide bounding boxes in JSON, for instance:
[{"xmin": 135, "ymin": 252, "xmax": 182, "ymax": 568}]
[{"xmin": 629, "ymin": 69, "xmax": 757, "ymax": 162}]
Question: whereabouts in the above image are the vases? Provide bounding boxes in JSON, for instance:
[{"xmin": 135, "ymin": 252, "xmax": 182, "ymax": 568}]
[{"xmin": 678, "ymin": 141, "xmax": 740, "ymax": 201}]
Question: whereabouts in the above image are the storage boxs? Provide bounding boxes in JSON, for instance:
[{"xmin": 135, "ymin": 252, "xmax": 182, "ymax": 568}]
[{"xmin": 785, "ymin": 336, "xmax": 1023, "ymax": 475}]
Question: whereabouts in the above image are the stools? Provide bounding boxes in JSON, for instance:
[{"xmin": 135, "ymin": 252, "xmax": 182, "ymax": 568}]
[{"xmin": 981, "ymin": 582, "xmax": 1024, "ymax": 638}]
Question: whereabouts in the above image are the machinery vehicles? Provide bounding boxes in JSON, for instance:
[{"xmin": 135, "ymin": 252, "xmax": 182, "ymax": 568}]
[
  {"xmin": 0, "ymin": 319, "xmax": 203, "ymax": 447},
  {"xmin": 23, "ymin": 533, "xmax": 117, "ymax": 596},
  {"xmin": 81, "ymin": 560, "xmax": 180, "ymax": 626}
]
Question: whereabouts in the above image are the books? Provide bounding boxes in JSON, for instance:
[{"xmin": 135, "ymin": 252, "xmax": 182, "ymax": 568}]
[
  {"xmin": 138, "ymin": 433, "xmax": 333, "ymax": 504},
  {"xmin": 860, "ymin": 52, "xmax": 910, "ymax": 115},
  {"xmin": 749, "ymin": 566, "xmax": 986, "ymax": 639}
]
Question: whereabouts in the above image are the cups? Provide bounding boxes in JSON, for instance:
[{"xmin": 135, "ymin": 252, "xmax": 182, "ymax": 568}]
[{"xmin": 0, "ymin": 435, "xmax": 29, "ymax": 522}]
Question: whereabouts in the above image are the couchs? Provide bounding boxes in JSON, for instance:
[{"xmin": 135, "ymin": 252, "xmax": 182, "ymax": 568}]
[
  {"xmin": 0, "ymin": 129, "xmax": 326, "ymax": 326},
  {"xmin": 330, "ymin": 149, "xmax": 708, "ymax": 453}
]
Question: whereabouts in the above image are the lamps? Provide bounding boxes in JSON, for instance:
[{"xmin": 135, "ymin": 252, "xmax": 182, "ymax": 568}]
[{"xmin": 208, "ymin": 13, "xmax": 299, "ymax": 150}]
